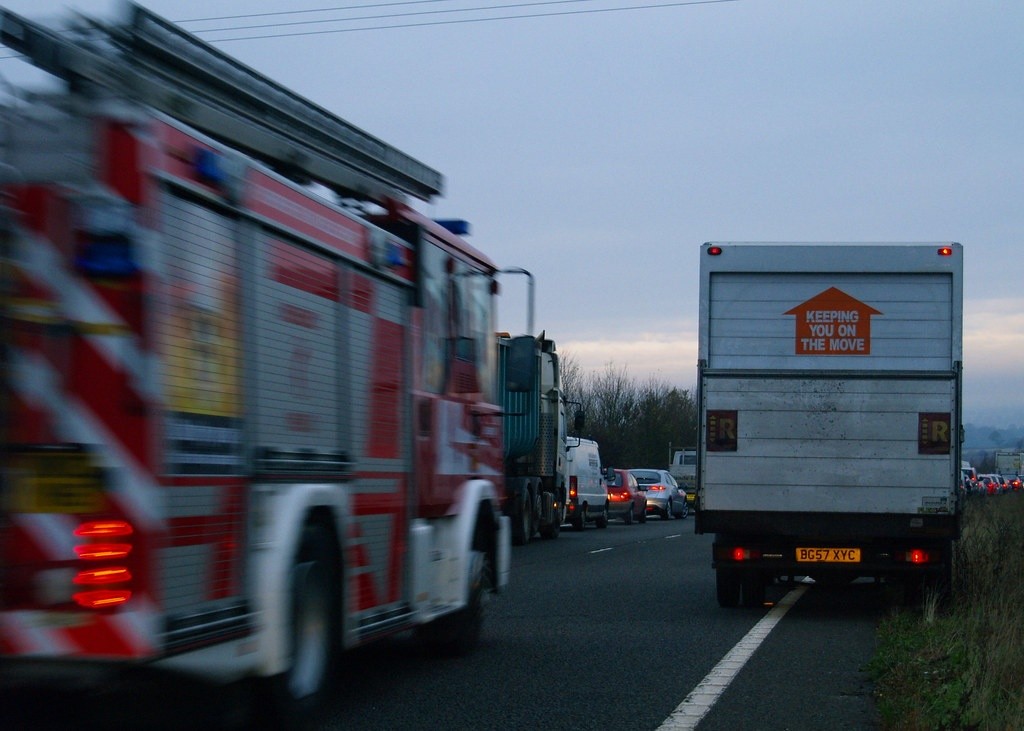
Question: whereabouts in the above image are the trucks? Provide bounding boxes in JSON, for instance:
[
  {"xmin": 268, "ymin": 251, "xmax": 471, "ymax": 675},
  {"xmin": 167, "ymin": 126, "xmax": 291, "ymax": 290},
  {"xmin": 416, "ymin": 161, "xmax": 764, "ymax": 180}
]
[
  {"xmin": 692, "ymin": 238, "xmax": 965, "ymax": 613},
  {"xmin": 563, "ymin": 437, "xmax": 617, "ymax": 531},
  {"xmin": 496, "ymin": 329, "xmax": 586, "ymax": 545},
  {"xmin": 996, "ymin": 452, "xmax": 1023, "ymax": 477},
  {"xmin": 669, "ymin": 451, "xmax": 696, "ymax": 509}
]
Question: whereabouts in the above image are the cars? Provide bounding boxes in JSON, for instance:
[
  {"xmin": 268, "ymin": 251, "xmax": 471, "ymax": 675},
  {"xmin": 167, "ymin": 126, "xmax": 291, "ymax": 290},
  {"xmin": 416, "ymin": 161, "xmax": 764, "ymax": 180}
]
[
  {"xmin": 627, "ymin": 468, "xmax": 689, "ymax": 521},
  {"xmin": 962, "ymin": 461, "xmax": 1018, "ymax": 497},
  {"xmin": 603, "ymin": 469, "xmax": 647, "ymax": 524}
]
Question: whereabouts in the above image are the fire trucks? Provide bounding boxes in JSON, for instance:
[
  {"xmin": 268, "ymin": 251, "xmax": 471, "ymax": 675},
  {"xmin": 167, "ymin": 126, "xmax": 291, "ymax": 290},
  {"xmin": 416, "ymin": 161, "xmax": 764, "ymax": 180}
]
[{"xmin": 0, "ymin": 2, "xmax": 540, "ymax": 723}]
